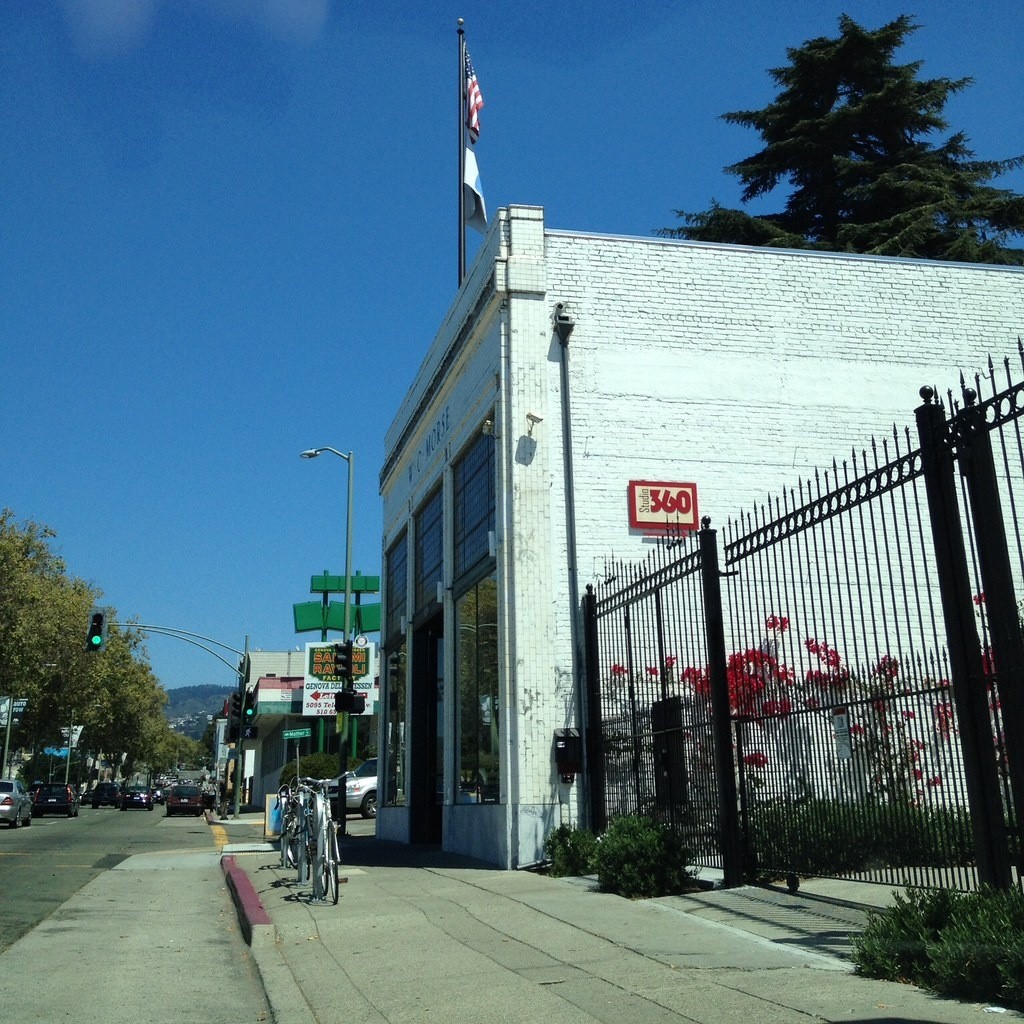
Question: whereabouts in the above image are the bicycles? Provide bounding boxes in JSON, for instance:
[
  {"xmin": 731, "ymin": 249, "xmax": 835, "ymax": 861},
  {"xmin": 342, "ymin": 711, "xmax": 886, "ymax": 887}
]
[{"xmin": 274, "ymin": 776, "xmax": 341, "ymax": 904}]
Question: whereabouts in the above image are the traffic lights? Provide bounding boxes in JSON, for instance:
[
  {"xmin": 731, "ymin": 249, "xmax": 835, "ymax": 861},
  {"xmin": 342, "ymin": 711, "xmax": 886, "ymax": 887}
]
[
  {"xmin": 232, "ymin": 693, "xmax": 254, "ymax": 719},
  {"xmin": 85, "ymin": 608, "xmax": 109, "ymax": 652}
]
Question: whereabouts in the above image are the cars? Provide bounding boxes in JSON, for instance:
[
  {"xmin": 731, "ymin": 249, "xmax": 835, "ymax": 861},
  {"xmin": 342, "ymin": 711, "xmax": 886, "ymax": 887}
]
[
  {"xmin": 33, "ymin": 783, "xmax": 81, "ymax": 817},
  {"xmin": 164, "ymin": 785, "xmax": 202, "ymax": 817},
  {"xmin": 81, "ymin": 781, "xmax": 164, "ymax": 811},
  {"xmin": 333, "ymin": 758, "xmax": 377, "ymax": 819},
  {"xmin": 0, "ymin": 779, "xmax": 33, "ymax": 828}
]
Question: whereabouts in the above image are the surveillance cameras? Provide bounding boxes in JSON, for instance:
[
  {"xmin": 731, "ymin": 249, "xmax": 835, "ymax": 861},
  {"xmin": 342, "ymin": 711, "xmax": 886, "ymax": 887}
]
[
  {"xmin": 555, "ymin": 303, "xmax": 563, "ymax": 311},
  {"xmin": 526, "ymin": 413, "xmax": 543, "ymax": 424}
]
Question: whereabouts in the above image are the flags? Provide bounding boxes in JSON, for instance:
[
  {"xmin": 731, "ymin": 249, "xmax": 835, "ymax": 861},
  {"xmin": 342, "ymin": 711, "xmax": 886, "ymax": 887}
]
[{"xmin": 463, "ymin": 40, "xmax": 484, "ymax": 145}]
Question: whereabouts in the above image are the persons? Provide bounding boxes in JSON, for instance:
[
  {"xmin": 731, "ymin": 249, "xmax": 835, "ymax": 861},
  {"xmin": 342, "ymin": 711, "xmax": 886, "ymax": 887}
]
[
  {"xmin": 208, "ymin": 784, "xmax": 216, "ymax": 813},
  {"xmin": 219, "ymin": 782, "xmax": 229, "ymax": 820}
]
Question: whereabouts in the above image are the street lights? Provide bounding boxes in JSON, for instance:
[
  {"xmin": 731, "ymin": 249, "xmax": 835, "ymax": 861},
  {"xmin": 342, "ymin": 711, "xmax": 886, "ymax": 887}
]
[{"xmin": 301, "ymin": 447, "xmax": 354, "ymax": 840}]
